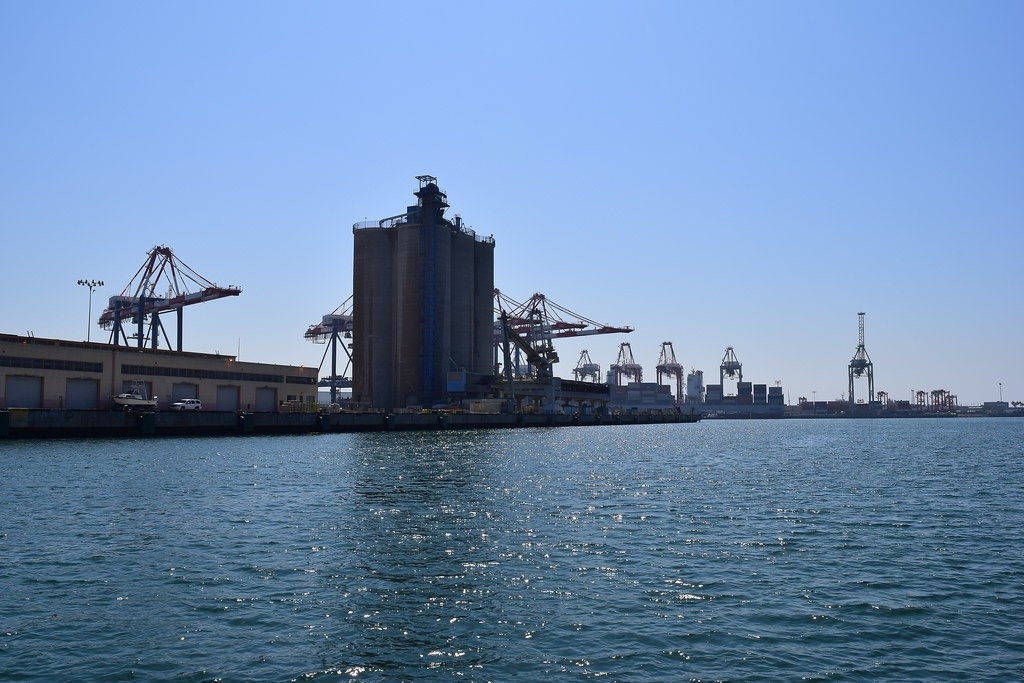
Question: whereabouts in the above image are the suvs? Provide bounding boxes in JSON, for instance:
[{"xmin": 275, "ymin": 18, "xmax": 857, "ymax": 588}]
[{"xmin": 171, "ymin": 398, "xmax": 203, "ymax": 412}]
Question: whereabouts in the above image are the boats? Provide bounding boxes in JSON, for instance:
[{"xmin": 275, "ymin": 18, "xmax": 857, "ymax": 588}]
[{"xmin": 112, "ymin": 393, "xmax": 159, "ymax": 413}]
